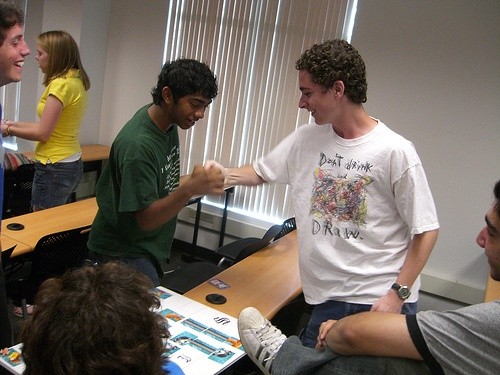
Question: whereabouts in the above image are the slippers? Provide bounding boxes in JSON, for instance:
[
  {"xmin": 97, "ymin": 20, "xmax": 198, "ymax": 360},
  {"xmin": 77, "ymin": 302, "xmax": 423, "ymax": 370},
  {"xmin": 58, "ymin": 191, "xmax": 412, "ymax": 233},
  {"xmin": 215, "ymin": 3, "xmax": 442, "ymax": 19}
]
[{"xmin": 12, "ymin": 304, "xmax": 36, "ymax": 317}]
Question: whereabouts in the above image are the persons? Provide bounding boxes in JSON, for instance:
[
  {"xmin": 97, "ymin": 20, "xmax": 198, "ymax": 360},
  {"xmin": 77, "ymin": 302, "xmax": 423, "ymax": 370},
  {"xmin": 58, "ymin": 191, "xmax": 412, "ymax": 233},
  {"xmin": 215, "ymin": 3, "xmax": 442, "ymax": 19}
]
[
  {"xmin": 207, "ymin": 39, "xmax": 440, "ymax": 348},
  {"xmin": 0, "ymin": 0, "xmax": 224, "ymax": 375},
  {"xmin": 237, "ymin": 182, "xmax": 500, "ymax": 375}
]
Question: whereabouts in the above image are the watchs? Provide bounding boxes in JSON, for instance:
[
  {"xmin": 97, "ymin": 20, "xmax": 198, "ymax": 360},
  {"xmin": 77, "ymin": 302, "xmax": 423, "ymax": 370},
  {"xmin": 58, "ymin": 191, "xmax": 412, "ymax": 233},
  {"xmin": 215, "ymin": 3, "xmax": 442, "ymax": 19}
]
[{"xmin": 392, "ymin": 283, "xmax": 411, "ymax": 300}]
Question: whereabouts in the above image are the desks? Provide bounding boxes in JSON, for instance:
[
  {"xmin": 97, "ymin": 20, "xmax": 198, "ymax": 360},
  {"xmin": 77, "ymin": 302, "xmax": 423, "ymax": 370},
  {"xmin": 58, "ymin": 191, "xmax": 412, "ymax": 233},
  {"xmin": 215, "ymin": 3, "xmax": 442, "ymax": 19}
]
[
  {"xmin": 22, "ymin": 144, "xmax": 111, "ymax": 203},
  {"xmin": 182, "ymin": 230, "xmax": 302, "ymax": 319},
  {"xmin": 0, "ymin": 183, "xmax": 235, "ymax": 263}
]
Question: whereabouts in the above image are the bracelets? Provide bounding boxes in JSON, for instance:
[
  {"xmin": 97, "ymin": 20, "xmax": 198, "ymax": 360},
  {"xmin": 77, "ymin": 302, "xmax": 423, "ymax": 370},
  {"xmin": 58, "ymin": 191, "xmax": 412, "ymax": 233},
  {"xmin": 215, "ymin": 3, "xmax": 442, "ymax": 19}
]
[{"xmin": 7, "ymin": 126, "xmax": 12, "ymax": 136}]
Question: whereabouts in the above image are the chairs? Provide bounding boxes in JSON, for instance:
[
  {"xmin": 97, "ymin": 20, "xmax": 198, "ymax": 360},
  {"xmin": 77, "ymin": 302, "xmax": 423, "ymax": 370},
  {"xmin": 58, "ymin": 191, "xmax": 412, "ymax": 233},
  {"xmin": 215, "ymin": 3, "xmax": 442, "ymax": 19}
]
[
  {"xmin": 216, "ymin": 217, "xmax": 297, "ymax": 267},
  {"xmin": 10, "ymin": 225, "xmax": 91, "ymax": 320}
]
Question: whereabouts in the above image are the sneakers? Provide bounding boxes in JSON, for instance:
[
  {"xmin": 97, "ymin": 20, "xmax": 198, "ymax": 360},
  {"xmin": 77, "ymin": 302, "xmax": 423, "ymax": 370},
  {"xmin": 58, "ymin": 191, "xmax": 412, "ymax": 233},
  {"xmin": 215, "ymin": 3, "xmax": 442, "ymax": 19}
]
[{"xmin": 238, "ymin": 306, "xmax": 289, "ymax": 375}]
